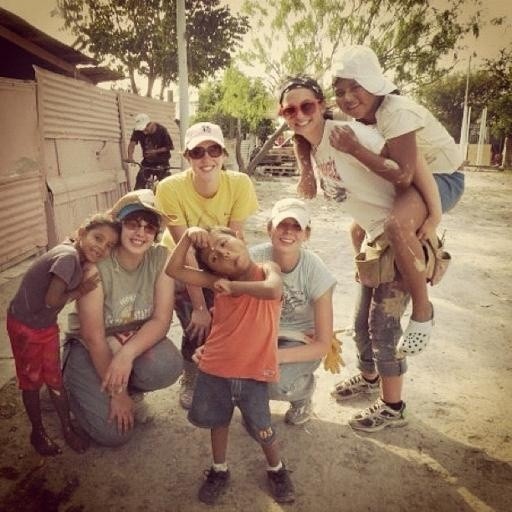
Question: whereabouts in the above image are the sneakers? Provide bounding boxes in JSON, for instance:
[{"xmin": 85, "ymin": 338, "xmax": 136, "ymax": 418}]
[
  {"xmin": 197, "ymin": 465, "xmax": 230, "ymax": 505},
  {"xmin": 348, "ymin": 401, "xmax": 408, "ymax": 434},
  {"xmin": 267, "ymin": 465, "xmax": 298, "ymax": 506},
  {"xmin": 131, "ymin": 391, "xmax": 155, "ymax": 424},
  {"xmin": 285, "ymin": 398, "xmax": 313, "ymax": 425},
  {"xmin": 179, "ymin": 371, "xmax": 198, "ymax": 409},
  {"xmin": 330, "ymin": 374, "xmax": 381, "ymax": 403}
]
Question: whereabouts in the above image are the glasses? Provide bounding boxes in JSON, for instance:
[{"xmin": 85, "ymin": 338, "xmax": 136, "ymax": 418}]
[
  {"xmin": 279, "ymin": 100, "xmax": 319, "ymax": 119},
  {"xmin": 124, "ymin": 217, "xmax": 158, "ymax": 235},
  {"xmin": 189, "ymin": 144, "xmax": 223, "ymax": 159}
]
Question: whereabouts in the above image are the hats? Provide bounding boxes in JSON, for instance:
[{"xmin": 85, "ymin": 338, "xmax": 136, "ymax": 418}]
[
  {"xmin": 134, "ymin": 114, "xmax": 150, "ymax": 131},
  {"xmin": 329, "ymin": 44, "xmax": 398, "ymax": 96},
  {"xmin": 270, "ymin": 197, "xmax": 312, "ymax": 230},
  {"xmin": 110, "ymin": 189, "xmax": 178, "ymax": 243},
  {"xmin": 183, "ymin": 121, "xmax": 225, "ymax": 150}
]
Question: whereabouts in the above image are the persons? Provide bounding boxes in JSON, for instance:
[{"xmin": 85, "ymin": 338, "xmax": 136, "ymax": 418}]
[
  {"xmin": 154, "ymin": 120, "xmax": 263, "ymax": 411},
  {"xmin": 245, "ymin": 198, "xmax": 339, "ymax": 428},
  {"xmin": 126, "ymin": 114, "xmax": 175, "ymax": 190},
  {"xmin": 6, "ymin": 214, "xmax": 120, "ymax": 457},
  {"xmin": 292, "ymin": 46, "xmax": 465, "ymax": 358},
  {"xmin": 277, "ymin": 76, "xmax": 452, "ymax": 433},
  {"xmin": 60, "ymin": 189, "xmax": 184, "ymax": 448},
  {"xmin": 163, "ymin": 223, "xmax": 297, "ymax": 505}
]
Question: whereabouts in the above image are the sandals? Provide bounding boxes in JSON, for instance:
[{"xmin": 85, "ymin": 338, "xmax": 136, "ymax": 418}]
[{"xmin": 396, "ymin": 302, "xmax": 439, "ymax": 357}]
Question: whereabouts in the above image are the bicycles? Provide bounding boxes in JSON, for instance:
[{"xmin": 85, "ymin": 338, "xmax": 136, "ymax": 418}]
[{"xmin": 118, "ymin": 157, "xmax": 187, "ymax": 193}]
